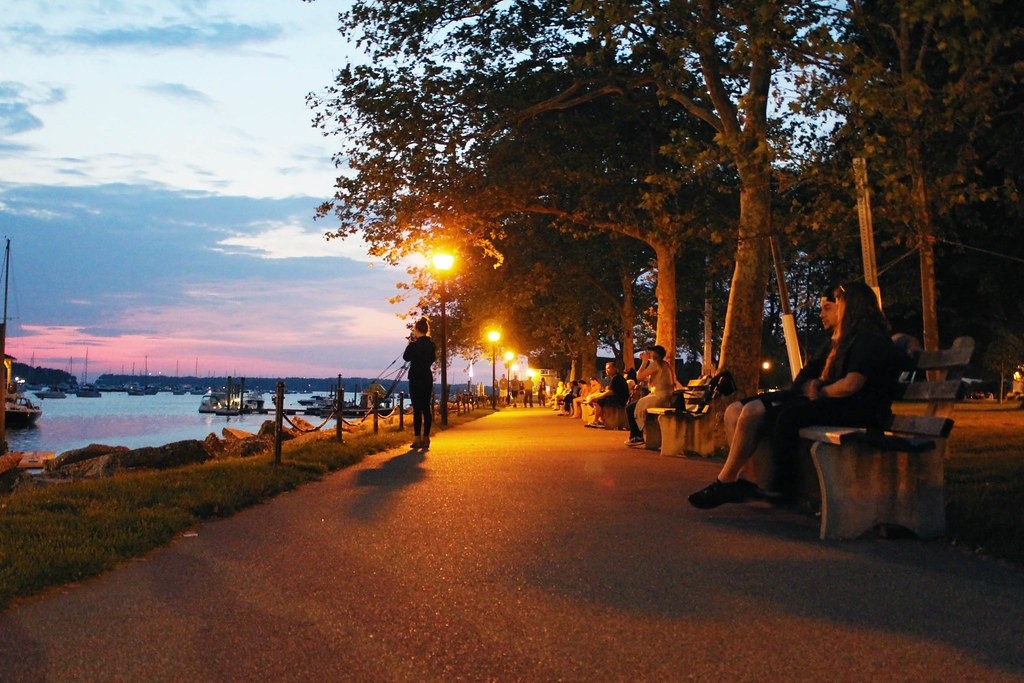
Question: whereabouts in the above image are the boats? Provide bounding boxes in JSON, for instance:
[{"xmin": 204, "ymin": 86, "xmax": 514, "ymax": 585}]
[
  {"xmin": 298, "ymin": 399, "xmax": 319, "ymax": 405},
  {"xmin": 5, "ymin": 353, "xmax": 42, "ymax": 424},
  {"xmin": 34, "ymin": 388, "xmax": 67, "ymax": 398},
  {"xmin": 77, "ymin": 389, "xmax": 101, "ymax": 397},
  {"xmin": 128, "ymin": 387, "xmax": 158, "ymax": 395},
  {"xmin": 198, "ymin": 400, "xmax": 220, "ymax": 412},
  {"xmin": 215, "ymin": 408, "xmax": 239, "ymax": 417},
  {"xmin": 174, "ymin": 389, "xmax": 185, "ymax": 395}
]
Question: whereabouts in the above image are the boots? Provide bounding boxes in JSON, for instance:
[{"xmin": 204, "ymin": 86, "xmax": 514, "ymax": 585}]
[{"xmin": 411, "ymin": 434, "xmax": 430, "ymax": 450}]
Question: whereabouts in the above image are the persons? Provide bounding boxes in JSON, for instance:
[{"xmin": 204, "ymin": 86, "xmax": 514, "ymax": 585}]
[
  {"xmin": 546, "ymin": 362, "xmax": 651, "ymax": 429},
  {"xmin": 498, "ymin": 373, "xmax": 509, "ymax": 407},
  {"xmin": 623, "ymin": 344, "xmax": 677, "ymax": 446},
  {"xmin": 688, "ymin": 278, "xmax": 896, "ymax": 519},
  {"xmin": 537, "ymin": 377, "xmax": 546, "ymax": 408},
  {"xmin": 402, "ymin": 315, "xmax": 437, "ymax": 447},
  {"xmin": 523, "ymin": 376, "xmax": 534, "ymax": 407},
  {"xmin": 511, "ymin": 375, "xmax": 520, "ymax": 407}
]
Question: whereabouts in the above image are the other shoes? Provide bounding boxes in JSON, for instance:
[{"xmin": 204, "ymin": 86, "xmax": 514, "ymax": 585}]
[
  {"xmin": 551, "ymin": 407, "xmax": 571, "ymax": 415},
  {"xmin": 570, "ymin": 414, "xmax": 580, "ymax": 419},
  {"xmin": 624, "ymin": 438, "xmax": 645, "ymax": 445},
  {"xmin": 589, "ymin": 408, "xmax": 596, "ymax": 416},
  {"xmin": 500, "ymin": 404, "xmax": 546, "ymax": 408},
  {"xmin": 584, "ymin": 421, "xmax": 606, "ymax": 428}
]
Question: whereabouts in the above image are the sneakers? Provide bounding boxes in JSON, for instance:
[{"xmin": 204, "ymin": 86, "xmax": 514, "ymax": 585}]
[{"xmin": 688, "ymin": 478, "xmax": 774, "ymax": 509}]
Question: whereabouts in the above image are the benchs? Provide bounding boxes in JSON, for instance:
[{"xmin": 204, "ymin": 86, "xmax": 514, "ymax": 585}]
[
  {"xmin": 747, "ymin": 333, "xmax": 974, "ymax": 539},
  {"xmin": 644, "ymin": 374, "xmax": 725, "ymax": 457},
  {"xmin": 554, "ymin": 385, "xmax": 641, "ymax": 430}
]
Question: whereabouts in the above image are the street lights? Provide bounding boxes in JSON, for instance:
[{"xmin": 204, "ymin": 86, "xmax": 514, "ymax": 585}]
[
  {"xmin": 431, "ymin": 247, "xmax": 458, "ymax": 426},
  {"xmin": 512, "ymin": 364, "xmax": 519, "ymax": 404},
  {"xmin": 487, "ymin": 329, "xmax": 501, "ymax": 408},
  {"xmin": 504, "ymin": 351, "xmax": 515, "ymax": 404}
]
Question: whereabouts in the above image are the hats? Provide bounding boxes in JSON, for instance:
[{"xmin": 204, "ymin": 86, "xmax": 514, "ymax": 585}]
[
  {"xmin": 647, "ymin": 345, "xmax": 666, "ymax": 358},
  {"xmin": 821, "ymin": 287, "xmax": 836, "ymax": 303}
]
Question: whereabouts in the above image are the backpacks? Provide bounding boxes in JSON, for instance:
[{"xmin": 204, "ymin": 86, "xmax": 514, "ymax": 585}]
[{"xmin": 684, "ymin": 369, "xmax": 737, "ymax": 415}]
